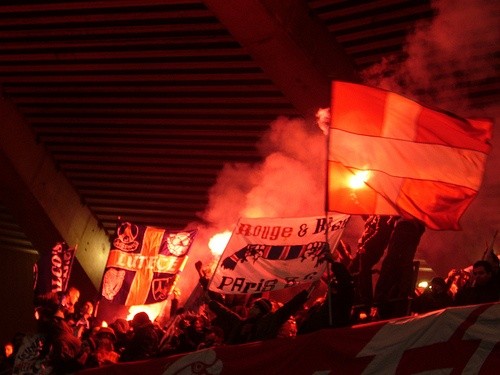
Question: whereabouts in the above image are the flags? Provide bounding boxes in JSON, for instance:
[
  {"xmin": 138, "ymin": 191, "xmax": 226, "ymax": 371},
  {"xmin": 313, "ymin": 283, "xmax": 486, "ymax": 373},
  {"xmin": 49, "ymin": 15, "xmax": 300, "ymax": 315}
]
[
  {"xmin": 32, "ymin": 239, "xmax": 76, "ymax": 293},
  {"xmin": 326, "ymin": 80, "xmax": 495, "ymax": 231},
  {"xmin": 100, "ymin": 218, "xmax": 199, "ymax": 305},
  {"xmin": 208, "ymin": 214, "xmax": 351, "ymax": 293}
]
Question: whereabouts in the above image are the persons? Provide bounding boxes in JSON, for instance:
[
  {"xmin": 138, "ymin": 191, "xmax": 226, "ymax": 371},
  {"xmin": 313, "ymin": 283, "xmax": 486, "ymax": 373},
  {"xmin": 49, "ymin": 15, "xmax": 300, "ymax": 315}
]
[{"xmin": 0, "ymin": 216, "xmax": 500, "ymax": 375}]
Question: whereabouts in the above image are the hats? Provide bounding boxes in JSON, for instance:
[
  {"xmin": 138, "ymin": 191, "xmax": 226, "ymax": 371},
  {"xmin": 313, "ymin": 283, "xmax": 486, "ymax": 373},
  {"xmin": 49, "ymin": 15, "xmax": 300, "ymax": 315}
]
[
  {"xmin": 96, "ymin": 327, "xmax": 117, "ymax": 340},
  {"xmin": 108, "ymin": 319, "xmax": 129, "ymax": 335},
  {"xmin": 257, "ymin": 298, "xmax": 272, "ymax": 313},
  {"xmin": 132, "ymin": 312, "xmax": 154, "ymax": 330}
]
